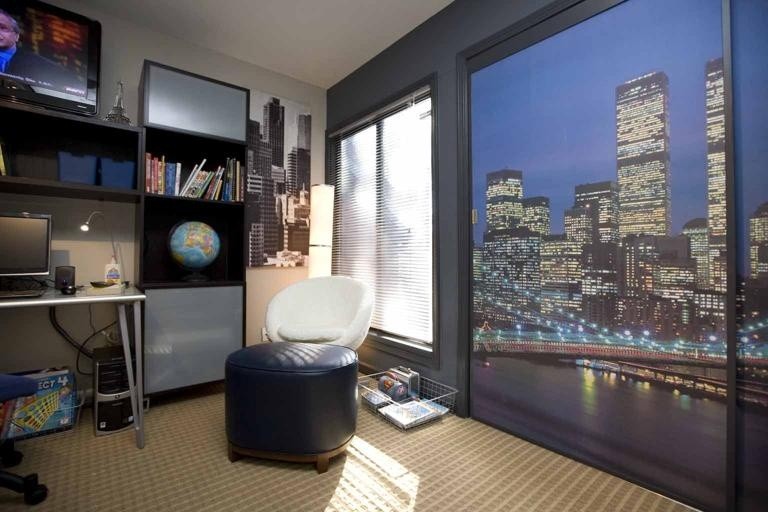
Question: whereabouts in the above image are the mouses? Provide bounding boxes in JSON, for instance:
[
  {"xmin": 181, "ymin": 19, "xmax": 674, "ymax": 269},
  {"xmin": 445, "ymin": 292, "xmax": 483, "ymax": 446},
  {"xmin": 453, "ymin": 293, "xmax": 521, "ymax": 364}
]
[{"xmin": 60, "ymin": 286, "xmax": 77, "ymax": 295}]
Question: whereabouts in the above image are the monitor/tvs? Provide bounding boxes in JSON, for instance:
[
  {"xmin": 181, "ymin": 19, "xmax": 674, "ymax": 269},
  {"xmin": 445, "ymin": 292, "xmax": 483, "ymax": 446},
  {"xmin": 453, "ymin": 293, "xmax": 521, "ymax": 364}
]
[
  {"xmin": 143, "ymin": 58, "xmax": 250, "ymax": 147},
  {"xmin": 0, "ymin": 212, "xmax": 52, "ymax": 277},
  {"xmin": 0, "ymin": 0, "xmax": 102, "ymax": 117}
]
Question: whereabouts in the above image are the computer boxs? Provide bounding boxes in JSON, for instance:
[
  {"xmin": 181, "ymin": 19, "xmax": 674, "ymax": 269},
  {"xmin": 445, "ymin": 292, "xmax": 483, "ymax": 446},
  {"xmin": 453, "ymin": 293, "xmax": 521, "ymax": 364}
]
[{"xmin": 92, "ymin": 346, "xmax": 138, "ymax": 436}]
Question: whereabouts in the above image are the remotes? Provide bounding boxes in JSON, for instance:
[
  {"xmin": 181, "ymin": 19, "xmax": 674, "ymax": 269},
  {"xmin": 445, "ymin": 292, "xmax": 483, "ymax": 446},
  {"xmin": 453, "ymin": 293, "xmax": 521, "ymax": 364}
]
[{"xmin": 90, "ymin": 281, "xmax": 116, "ymax": 288}]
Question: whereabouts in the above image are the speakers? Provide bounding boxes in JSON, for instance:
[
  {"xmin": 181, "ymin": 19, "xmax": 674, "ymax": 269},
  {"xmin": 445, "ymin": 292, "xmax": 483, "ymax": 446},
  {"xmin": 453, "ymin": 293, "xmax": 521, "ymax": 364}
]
[{"xmin": 55, "ymin": 266, "xmax": 75, "ymax": 289}]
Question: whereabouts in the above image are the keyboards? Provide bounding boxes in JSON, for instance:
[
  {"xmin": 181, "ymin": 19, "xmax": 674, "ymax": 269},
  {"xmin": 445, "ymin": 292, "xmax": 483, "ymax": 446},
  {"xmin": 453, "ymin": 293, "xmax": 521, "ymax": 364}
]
[{"xmin": 0, "ymin": 289, "xmax": 44, "ymax": 299}]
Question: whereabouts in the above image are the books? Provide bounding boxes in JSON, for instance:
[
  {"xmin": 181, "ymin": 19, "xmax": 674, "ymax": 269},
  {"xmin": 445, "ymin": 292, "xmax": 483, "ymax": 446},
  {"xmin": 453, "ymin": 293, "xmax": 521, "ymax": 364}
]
[
  {"xmin": 146, "ymin": 151, "xmax": 244, "ymax": 203},
  {"xmin": 377, "ymin": 395, "xmax": 450, "ymax": 430}
]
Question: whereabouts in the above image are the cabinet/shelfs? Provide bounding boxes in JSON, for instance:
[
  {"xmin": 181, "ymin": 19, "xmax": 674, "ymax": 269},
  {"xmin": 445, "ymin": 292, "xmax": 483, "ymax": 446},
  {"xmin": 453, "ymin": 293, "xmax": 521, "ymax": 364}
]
[
  {"xmin": 0, "ymin": 101, "xmax": 146, "ymax": 202},
  {"xmin": 134, "ymin": 59, "xmax": 251, "ymax": 407}
]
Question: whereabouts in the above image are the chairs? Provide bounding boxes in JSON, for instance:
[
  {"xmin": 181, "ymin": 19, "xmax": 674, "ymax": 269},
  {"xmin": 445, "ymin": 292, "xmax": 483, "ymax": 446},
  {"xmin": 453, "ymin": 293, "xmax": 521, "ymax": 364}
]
[{"xmin": 225, "ymin": 275, "xmax": 373, "ymax": 473}]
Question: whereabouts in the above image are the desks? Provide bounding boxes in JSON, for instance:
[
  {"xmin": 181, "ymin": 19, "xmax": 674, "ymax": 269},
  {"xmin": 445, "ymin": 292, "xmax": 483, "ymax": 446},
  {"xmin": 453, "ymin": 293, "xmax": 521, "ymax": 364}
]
[{"xmin": 0, "ymin": 284, "xmax": 146, "ymax": 449}]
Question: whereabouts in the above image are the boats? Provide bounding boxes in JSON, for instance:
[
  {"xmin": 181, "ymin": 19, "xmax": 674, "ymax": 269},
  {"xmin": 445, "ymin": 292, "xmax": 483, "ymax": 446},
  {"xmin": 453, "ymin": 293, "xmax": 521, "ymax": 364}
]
[{"xmin": 575, "ymin": 357, "xmax": 767, "ymax": 418}]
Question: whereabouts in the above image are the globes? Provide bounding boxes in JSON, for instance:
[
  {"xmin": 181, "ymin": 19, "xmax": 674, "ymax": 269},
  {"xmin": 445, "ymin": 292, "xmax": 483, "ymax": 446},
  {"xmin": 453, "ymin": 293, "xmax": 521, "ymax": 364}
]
[{"xmin": 167, "ymin": 219, "xmax": 221, "ymax": 282}]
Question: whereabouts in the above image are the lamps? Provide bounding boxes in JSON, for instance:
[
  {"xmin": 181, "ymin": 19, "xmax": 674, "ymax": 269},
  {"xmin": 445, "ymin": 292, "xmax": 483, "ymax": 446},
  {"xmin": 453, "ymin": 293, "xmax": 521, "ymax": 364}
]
[{"xmin": 308, "ymin": 184, "xmax": 335, "ymax": 278}]
